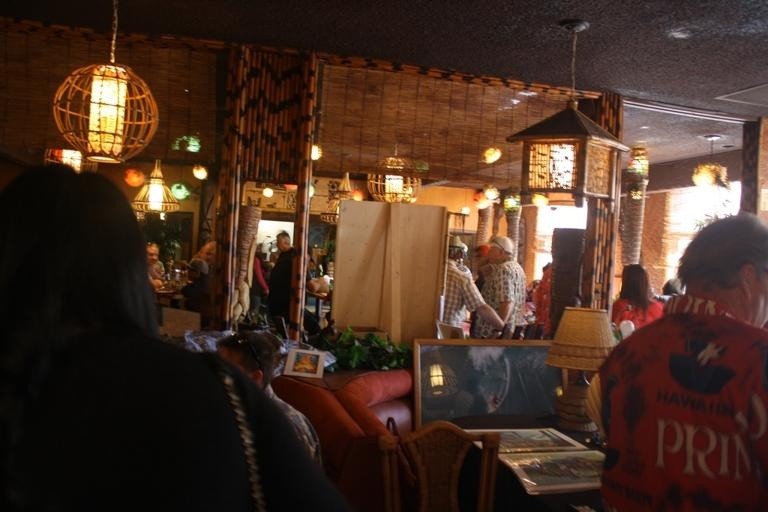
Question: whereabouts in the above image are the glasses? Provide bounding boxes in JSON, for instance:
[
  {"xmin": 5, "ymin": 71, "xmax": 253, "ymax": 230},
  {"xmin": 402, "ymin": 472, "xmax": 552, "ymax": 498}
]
[
  {"xmin": 234, "ymin": 331, "xmax": 265, "ymax": 372},
  {"xmin": 490, "ymin": 236, "xmax": 505, "ymax": 251}
]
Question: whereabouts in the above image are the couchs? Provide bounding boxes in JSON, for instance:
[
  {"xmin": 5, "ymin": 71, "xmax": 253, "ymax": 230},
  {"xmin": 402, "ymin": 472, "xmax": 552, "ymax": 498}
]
[{"xmin": 270, "ymin": 359, "xmax": 420, "ymax": 512}]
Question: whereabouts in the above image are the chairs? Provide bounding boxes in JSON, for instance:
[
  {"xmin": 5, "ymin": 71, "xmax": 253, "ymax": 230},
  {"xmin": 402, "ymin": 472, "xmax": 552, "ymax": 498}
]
[
  {"xmin": 160, "ymin": 305, "xmax": 201, "ymax": 339},
  {"xmin": 378, "ymin": 419, "xmax": 500, "ymax": 512}
]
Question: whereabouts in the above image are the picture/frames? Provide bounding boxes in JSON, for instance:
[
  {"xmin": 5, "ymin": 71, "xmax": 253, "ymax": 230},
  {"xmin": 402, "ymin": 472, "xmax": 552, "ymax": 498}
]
[
  {"xmin": 282, "ymin": 349, "xmax": 325, "ymax": 380},
  {"xmin": 413, "ymin": 337, "xmax": 569, "ymax": 429}
]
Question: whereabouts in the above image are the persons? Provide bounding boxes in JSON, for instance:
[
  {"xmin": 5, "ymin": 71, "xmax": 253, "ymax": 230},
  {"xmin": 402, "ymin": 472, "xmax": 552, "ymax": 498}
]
[
  {"xmin": 304, "ymin": 246, "xmax": 328, "ymax": 333},
  {"xmin": 267, "ymin": 231, "xmax": 294, "ymax": 324},
  {"xmin": 466, "ymin": 246, "xmax": 491, "ymax": 338},
  {"xmin": 654, "ymin": 277, "xmax": 685, "ymax": 303},
  {"xmin": 1, "ymin": 165, "xmax": 80, "ymax": 344},
  {"xmin": 145, "ymin": 242, "xmax": 165, "ymax": 281},
  {"xmin": 583, "ymin": 211, "xmax": 768, "ymax": 511},
  {"xmin": 215, "ymin": 330, "xmax": 326, "ymax": 480},
  {"xmin": 181, "ymin": 258, "xmax": 212, "ymax": 330},
  {"xmin": 440, "ymin": 235, "xmax": 505, "ymax": 339},
  {"xmin": 610, "ymin": 263, "xmax": 666, "ymax": 333},
  {"xmin": 534, "ymin": 260, "xmax": 552, "ymax": 340},
  {"xmin": 249, "ymin": 256, "xmax": 270, "ymax": 319},
  {"xmin": 475, "ymin": 234, "xmax": 527, "ymax": 340},
  {"xmin": 0, "ymin": 162, "xmax": 352, "ymax": 512}
]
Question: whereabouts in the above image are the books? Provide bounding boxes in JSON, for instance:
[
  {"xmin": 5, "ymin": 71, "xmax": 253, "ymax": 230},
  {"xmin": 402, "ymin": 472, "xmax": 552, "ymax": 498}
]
[{"xmin": 459, "ymin": 426, "xmax": 606, "ymax": 495}]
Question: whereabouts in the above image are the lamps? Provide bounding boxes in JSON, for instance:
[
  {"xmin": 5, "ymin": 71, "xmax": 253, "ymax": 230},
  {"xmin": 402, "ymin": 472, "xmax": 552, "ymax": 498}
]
[
  {"xmin": 319, "ymin": 172, "xmax": 355, "ymax": 226},
  {"xmin": 367, "ymin": 137, "xmax": 422, "ymax": 205},
  {"xmin": 694, "ymin": 141, "xmax": 731, "ymax": 191},
  {"xmin": 130, "ymin": 157, "xmax": 180, "ymax": 214},
  {"xmin": 506, "ymin": 32, "xmax": 631, "ymax": 214},
  {"xmin": 52, "ymin": 1, "xmax": 160, "ymax": 163},
  {"xmin": 545, "ymin": 307, "xmax": 621, "ymax": 447}
]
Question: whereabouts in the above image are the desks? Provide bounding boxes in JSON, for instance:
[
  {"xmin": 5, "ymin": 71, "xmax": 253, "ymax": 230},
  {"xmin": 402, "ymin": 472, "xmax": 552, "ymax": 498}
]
[
  {"xmin": 304, "ymin": 288, "xmax": 333, "ymax": 328},
  {"xmin": 152, "ymin": 284, "xmax": 182, "ymax": 294}
]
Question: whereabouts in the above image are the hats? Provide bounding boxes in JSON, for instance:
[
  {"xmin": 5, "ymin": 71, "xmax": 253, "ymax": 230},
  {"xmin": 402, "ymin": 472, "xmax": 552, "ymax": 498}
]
[
  {"xmin": 490, "ymin": 234, "xmax": 513, "ymax": 255},
  {"xmin": 449, "ymin": 236, "xmax": 468, "ymax": 253}
]
